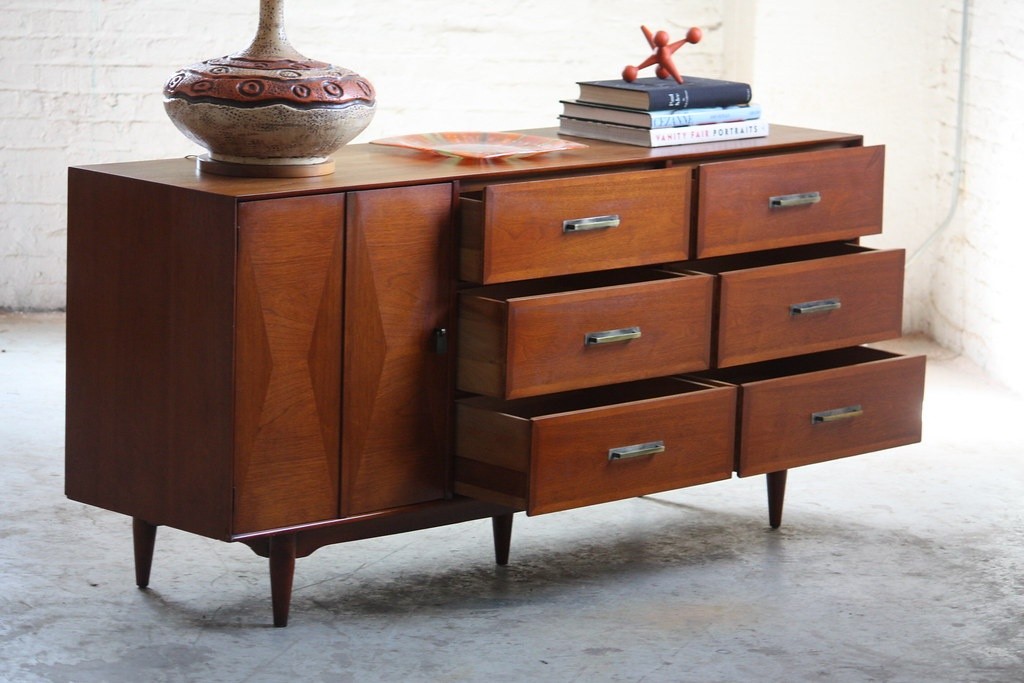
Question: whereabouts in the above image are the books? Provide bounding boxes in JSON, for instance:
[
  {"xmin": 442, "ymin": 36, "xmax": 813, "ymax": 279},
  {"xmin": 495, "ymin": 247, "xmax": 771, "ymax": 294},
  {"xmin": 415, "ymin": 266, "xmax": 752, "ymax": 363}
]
[{"xmin": 555, "ymin": 73, "xmax": 769, "ymax": 148}]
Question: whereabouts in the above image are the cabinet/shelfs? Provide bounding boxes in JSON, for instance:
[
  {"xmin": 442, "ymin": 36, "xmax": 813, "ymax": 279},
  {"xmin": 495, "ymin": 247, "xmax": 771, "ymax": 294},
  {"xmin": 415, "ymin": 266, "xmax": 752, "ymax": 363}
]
[{"xmin": 64, "ymin": 119, "xmax": 927, "ymax": 627}]
